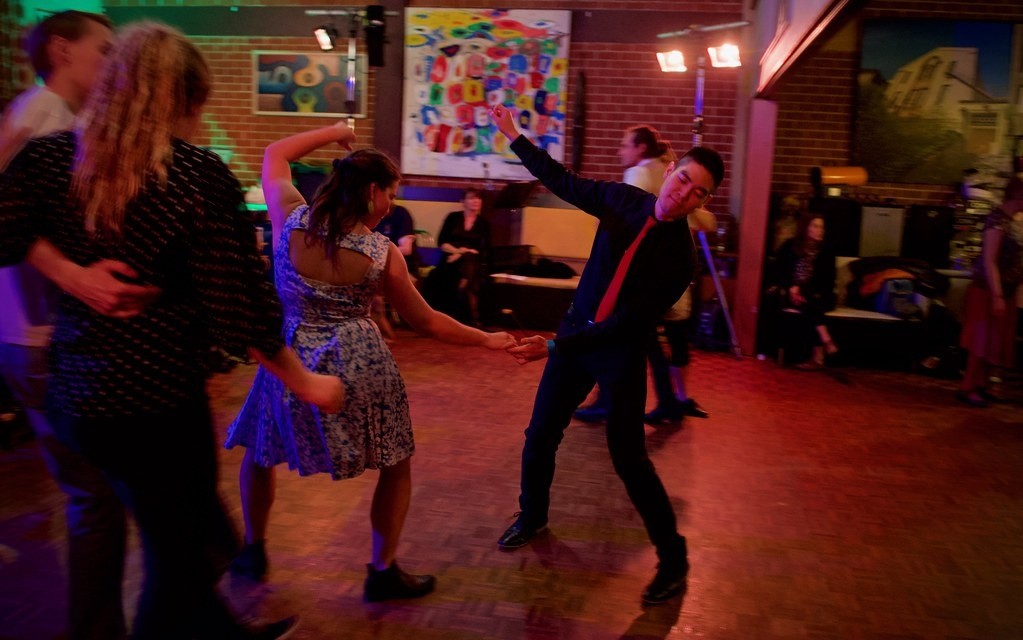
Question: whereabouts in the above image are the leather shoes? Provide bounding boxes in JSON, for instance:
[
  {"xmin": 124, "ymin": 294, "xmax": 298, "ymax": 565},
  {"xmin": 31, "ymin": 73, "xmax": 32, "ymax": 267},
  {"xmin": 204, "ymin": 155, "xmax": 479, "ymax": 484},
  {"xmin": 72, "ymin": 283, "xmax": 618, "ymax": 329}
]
[
  {"xmin": 228, "ymin": 537, "xmax": 267, "ymax": 579},
  {"xmin": 364, "ymin": 563, "xmax": 436, "ymax": 601},
  {"xmin": 497, "ymin": 511, "xmax": 547, "ymax": 548},
  {"xmin": 643, "ymin": 564, "xmax": 686, "ymax": 603}
]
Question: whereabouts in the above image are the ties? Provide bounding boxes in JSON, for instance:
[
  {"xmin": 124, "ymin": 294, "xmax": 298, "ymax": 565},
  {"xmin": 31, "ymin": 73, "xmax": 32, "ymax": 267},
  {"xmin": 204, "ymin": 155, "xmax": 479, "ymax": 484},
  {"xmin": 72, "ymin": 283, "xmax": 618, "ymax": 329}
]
[{"xmin": 594, "ymin": 215, "xmax": 656, "ymax": 323}]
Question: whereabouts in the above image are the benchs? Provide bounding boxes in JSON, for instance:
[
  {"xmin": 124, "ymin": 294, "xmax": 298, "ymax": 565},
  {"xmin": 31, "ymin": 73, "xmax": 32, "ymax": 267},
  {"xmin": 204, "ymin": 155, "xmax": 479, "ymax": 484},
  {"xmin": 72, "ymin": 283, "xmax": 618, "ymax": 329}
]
[
  {"xmin": 776, "ymin": 252, "xmax": 965, "ymax": 365},
  {"xmin": 391, "ymin": 197, "xmax": 601, "ymax": 329}
]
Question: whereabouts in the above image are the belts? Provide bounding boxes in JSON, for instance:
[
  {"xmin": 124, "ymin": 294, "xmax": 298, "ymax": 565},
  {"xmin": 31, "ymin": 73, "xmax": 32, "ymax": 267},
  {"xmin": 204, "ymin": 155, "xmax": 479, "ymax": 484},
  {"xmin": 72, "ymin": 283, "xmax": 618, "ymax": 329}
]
[{"xmin": 569, "ymin": 308, "xmax": 643, "ymax": 338}]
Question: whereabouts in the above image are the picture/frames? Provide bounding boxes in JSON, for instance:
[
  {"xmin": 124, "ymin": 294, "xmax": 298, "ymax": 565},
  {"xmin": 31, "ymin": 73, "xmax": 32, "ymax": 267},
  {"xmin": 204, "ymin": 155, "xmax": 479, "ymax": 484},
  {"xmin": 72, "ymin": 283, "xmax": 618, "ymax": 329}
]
[{"xmin": 251, "ymin": 50, "xmax": 368, "ymax": 120}]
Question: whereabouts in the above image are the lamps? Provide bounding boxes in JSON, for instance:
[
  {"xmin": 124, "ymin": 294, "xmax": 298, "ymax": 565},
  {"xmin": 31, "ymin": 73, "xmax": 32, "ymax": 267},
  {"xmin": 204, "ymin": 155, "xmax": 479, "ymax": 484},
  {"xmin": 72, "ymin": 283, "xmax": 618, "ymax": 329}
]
[{"xmin": 311, "ymin": 21, "xmax": 339, "ymax": 51}]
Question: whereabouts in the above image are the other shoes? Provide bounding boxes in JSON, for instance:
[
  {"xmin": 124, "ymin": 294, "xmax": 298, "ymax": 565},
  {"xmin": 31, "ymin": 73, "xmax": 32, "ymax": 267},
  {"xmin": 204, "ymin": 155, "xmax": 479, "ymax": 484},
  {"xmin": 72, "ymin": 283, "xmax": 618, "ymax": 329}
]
[
  {"xmin": 955, "ymin": 387, "xmax": 990, "ymax": 407},
  {"xmin": 679, "ymin": 398, "xmax": 707, "ymax": 417},
  {"xmin": 826, "ymin": 337, "xmax": 837, "ymax": 355},
  {"xmin": 795, "ymin": 360, "xmax": 826, "ymax": 370},
  {"xmin": 982, "ymin": 386, "xmax": 1010, "ymax": 404},
  {"xmin": 131, "ymin": 598, "xmax": 294, "ymax": 640},
  {"xmin": 574, "ymin": 400, "xmax": 608, "ymax": 423},
  {"xmin": 643, "ymin": 400, "xmax": 684, "ymax": 424}
]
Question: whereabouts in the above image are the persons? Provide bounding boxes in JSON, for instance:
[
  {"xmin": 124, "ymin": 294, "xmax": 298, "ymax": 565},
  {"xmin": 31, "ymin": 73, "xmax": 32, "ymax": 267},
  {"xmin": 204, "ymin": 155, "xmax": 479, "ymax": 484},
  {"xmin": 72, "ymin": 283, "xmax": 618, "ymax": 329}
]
[
  {"xmin": 779, "ymin": 212, "xmax": 851, "ymax": 373},
  {"xmin": 956, "ymin": 171, "xmax": 1023, "ymax": 406},
  {"xmin": 0, "ymin": 12, "xmax": 723, "ymax": 640}
]
